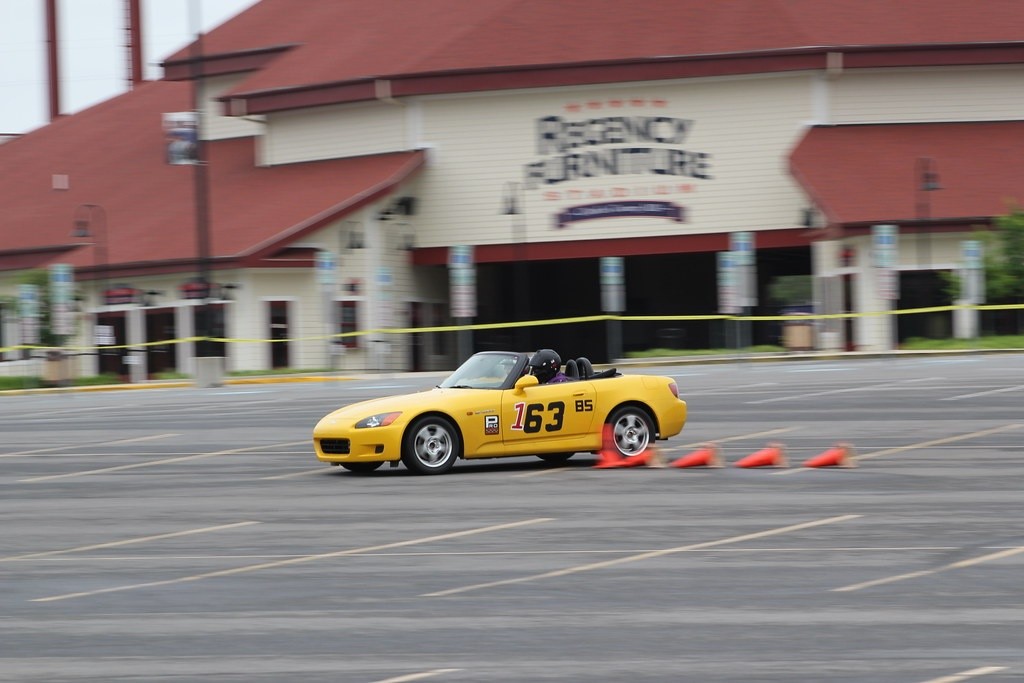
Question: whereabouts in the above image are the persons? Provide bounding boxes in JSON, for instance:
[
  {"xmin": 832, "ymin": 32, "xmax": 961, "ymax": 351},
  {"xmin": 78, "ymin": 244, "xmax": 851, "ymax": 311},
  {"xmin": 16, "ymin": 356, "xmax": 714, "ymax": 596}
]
[
  {"xmin": 528, "ymin": 348, "xmax": 569, "ymax": 385},
  {"xmin": 501, "ymin": 353, "xmax": 530, "ymax": 377}
]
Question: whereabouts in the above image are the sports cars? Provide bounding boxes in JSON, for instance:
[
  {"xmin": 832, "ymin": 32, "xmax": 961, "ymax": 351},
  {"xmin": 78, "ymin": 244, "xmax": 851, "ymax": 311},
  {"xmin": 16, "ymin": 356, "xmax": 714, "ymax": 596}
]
[{"xmin": 312, "ymin": 351, "xmax": 686, "ymax": 475}]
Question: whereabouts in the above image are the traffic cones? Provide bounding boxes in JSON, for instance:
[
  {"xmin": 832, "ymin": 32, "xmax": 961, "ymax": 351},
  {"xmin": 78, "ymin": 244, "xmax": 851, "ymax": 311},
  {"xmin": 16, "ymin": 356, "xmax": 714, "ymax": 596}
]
[
  {"xmin": 737, "ymin": 449, "xmax": 782, "ymax": 468},
  {"xmin": 593, "ymin": 449, "xmax": 653, "ymax": 468},
  {"xmin": 669, "ymin": 449, "xmax": 714, "ymax": 466},
  {"xmin": 802, "ymin": 449, "xmax": 849, "ymax": 466}
]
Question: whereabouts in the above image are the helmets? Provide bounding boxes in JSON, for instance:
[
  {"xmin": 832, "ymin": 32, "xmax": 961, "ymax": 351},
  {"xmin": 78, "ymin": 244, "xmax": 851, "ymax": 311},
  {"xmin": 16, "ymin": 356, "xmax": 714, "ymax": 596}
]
[{"xmin": 529, "ymin": 349, "xmax": 562, "ymax": 383}]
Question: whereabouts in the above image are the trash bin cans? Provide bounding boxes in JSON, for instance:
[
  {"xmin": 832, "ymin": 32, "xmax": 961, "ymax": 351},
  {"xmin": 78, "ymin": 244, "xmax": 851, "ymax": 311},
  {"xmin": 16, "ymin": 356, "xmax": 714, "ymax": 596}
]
[{"xmin": 780, "ymin": 305, "xmax": 814, "ymax": 349}]
